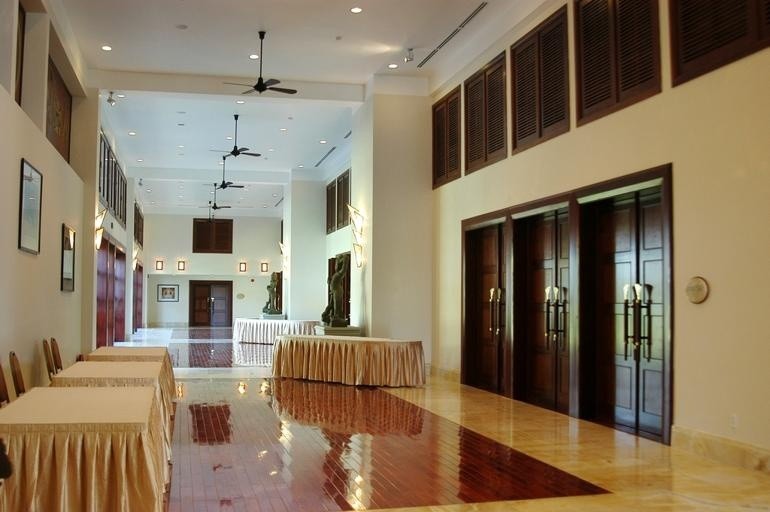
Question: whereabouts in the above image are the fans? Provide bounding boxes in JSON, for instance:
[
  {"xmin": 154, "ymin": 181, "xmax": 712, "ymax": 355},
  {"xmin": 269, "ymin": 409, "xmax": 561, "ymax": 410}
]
[
  {"xmin": 202, "ymin": 155, "xmax": 244, "ymax": 190},
  {"xmin": 198, "ymin": 182, "xmax": 231, "ymax": 210},
  {"xmin": 223, "ymin": 30, "xmax": 298, "ymax": 95},
  {"xmin": 208, "ymin": 114, "xmax": 262, "ymax": 157},
  {"xmin": 195, "ymin": 201, "xmax": 230, "ymax": 225}
]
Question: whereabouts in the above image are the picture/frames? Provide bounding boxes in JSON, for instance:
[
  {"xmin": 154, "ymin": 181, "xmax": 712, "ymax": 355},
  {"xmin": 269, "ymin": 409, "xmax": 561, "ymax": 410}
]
[
  {"xmin": 18, "ymin": 157, "xmax": 44, "ymax": 256},
  {"xmin": 156, "ymin": 284, "xmax": 179, "ymax": 302},
  {"xmin": 61, "ymin": 223, "xmax": 77, "ymax": 292}
]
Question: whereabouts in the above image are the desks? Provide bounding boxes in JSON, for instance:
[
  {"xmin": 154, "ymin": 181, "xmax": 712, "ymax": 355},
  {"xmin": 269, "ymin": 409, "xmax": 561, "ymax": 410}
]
[
  {"xmin": 51, "ymin": 361, "xmax": 176, "ymax": 465},
  {"xmin": 88, "ymin": 345, "xmax": 178, "ymax": 421},
  {"xmin": 0, "ymin": 387, "xmax": 173, "ymax": 512},
  {"xmin": 272, "ymin": 335, "xmax": 427, "ymax": 388},
  {"xmin": 234, "ymin": 318, "xmax": 322, "ymax": 345},
  {"xmin": 272, "ymin": 377, "xmax": 427, "ymax": 438}
]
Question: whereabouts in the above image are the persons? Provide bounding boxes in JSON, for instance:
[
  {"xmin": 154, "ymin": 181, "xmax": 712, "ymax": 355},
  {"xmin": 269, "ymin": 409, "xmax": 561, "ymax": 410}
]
[
  {"xmin": 262, "ymin": 273, "xmax": 278, "ymax": 312},
  {"xmin": 327, "ymin": 254, "xmax": 349, "ymax": 321}
]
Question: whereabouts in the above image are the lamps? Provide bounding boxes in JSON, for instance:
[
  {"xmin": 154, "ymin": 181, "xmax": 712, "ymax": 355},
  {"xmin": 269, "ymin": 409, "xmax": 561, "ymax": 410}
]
[
  {"xmin": 346, "ymin": 203, "xmax": 364, "ymax": 269},
  {"xmin": 404, "ymin": 48, "xmax": 414, "ymax": 62},
  {"xmin": 107, "ymin": 91, "xmax": 116, "ymax": 106},
  {"xmin": 96, "ymin": 208, "xmax": 109, "ymax": 251},
  {"xmin": 278, "ymin": 242, "xmax": 288, "ymax": 280}
]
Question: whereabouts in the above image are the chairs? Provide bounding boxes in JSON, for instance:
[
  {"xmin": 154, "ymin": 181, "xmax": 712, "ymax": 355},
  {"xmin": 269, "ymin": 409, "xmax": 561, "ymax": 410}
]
[
  {"xmin": 79, "ymin": 353, "xmax": 88, "ymax": 361},
  {"xmin": 50, "ymin": 337, "xmax": 63, "ymax": 373},
  {"xmin": 43, "ymin": 339, "xmax": 55, "ymax": 380},
  {"xmin": 0, "ymin": 364, "xmax": 10, "ymax": 409},
  {"xmin": 9, "ymin": 351, "xmax": 26, "ymax": 398}
]
